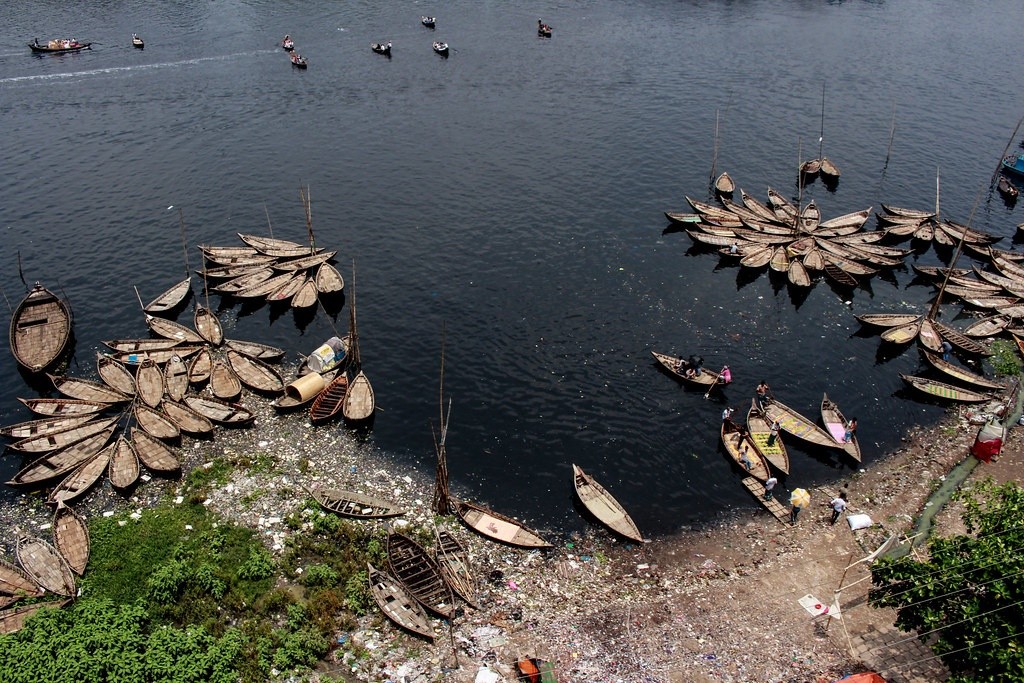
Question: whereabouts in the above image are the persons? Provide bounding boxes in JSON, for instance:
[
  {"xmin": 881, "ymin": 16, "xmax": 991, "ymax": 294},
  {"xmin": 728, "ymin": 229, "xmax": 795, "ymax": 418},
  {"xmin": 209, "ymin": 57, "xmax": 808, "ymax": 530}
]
[
  {"xmin": 756, "ymin": 380, "xmax": 769, "ymax": 406},
  {"xmin": 375, "ymin": 41, "xmax": 392, "ymax": 49},
  {"xmin": 764, "ymin": 474, "xmax": 777, "ymax": 499},
  {"xmin": 63, "ymin": 36, "xmax": 78, "ymax": 48},
  {"xmin": 839, "ymin": 483, "xmax": 848, "ymax": 499},
  {"xmin": 675, "ymin": 354, "xmax": 704, "ymax": 378},
  {"xmin": 791, "ymin": 506, "xmax": 800, "ymax": 526},
  {"xmin": 737, "ymin": 424, "xmax": 748, "ymax": 450},
  {"xmin": 831, "ymin": 493, "xmax": 846, "ymax": 525},
  {"xmin": 768, "ymin": 420, "xmax": 780, "ymax": 446},
  {"xmin": 132, "ymin": 32, "xmax": 137, "ymax": 40},
  {"xmin": 939, "ymin": 338, "xmax": 952, "ymax": 360},
  {"xmin": 720, "ymin": 365, "xmax": 731, "ymax": 383},
  {"xmin": 738, "ymin": 446, "xmax": 752, "ymax": 471},
  {"xmin": 848, "ymin": 417, "xmax": 858, "ymax": 438},
  {"xmin": 722, "ymin": 406, "xmax": 735, "ymax": 433},
  {"xmin": 425, "ymin": 16, "xmax": 434, "ymax": 22}
]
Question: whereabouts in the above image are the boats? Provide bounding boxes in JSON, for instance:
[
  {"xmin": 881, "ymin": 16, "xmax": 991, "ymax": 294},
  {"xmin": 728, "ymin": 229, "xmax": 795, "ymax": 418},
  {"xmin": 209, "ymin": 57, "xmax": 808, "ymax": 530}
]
[
  {"xmin": 366, "ymin": 527, "xmax": 481, "ymax": 638},
  {"xmin": 450, "ymin": 497, "xmax": 555, "ymax": 547},
  {"xmin": 852, "ymin": 222, "xmax": 1024, "ymax": 357},
  {"xmin": 923, "ymin": 349, "xmax": 1006, "ymax": 389},
  {"xmin": 875, "ymin": 202, "xmax": 1006, "ymax": 246},
  {"xmin": 0, "ymin": 232, "xmax": 375, "ymax": 503},
  {"xmin": 302, "ymin": 484, "xmax": 407, "ymax": 517},
  {"xmin": 282, "ymin": 34, "xmax": 294, "ymax": 49},
  {"xmin": 799, "ymin": 156, "xmax": 841, "ymax": 176},
  {"xmin": 9, "ymin": 281, "xmax": 71, "ymax": 372},
  {"xmin": 721, "ymin": 392, "xmax": 861, "ymax": 482},
  {"xmin": 1, "ymin": 497, "xmax": 91, "ymax": 636},
  {"xmin": 651, "ymin": 350, "xmax": 732, "ymax": 384},
  {"xmin": 715, "ymin": 172, "xmax": 735, "ymax": 192},
  {"xmin": 290, "ymin": 50, "xmax": 307, "ymax": 66},
  {"xmin": 421, "ymin": 16, "xmax": 434, "ymax": 25},
  {"xmin": 572, "ymin": 463, "xmax": 644, "ymax": 543},
  {"xmin": 664, "ymin": 186, "xmax": 915, "ymax": 287},
  {"xmin": 741, "ymin": 476, "xmax": 802, "ymax": 529},
  {"xmin": 27, "ymin": 39, "xmax": 92, "ymax": 51},
  {"xmin": 898, "ymin": 372, "xmax": 991, "ymax": 401},
  {"xmin": 132, "ymin": 36, "xmax": 144, "ymax": 47},
  {"xmin": 999, "ymin": 140, "xmax": 1024, "ymax": 197},
  {"xmin": 433, "ymin": 41, "xmax": 449, "ymax": 52},
  {"xmin": 372, "ymin": 43, "xmax": 389, "ymax": 53},
  {"xmin": 538, "ymin": 24, "xmax": 552, "ymax": 35}
]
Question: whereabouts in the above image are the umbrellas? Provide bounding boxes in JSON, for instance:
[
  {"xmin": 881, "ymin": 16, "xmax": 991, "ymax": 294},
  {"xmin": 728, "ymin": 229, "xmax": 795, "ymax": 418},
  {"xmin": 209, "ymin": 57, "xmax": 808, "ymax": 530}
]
[{"xmin": 791, "ymin": 488, "xmax": 810, "ymax": 508}]
